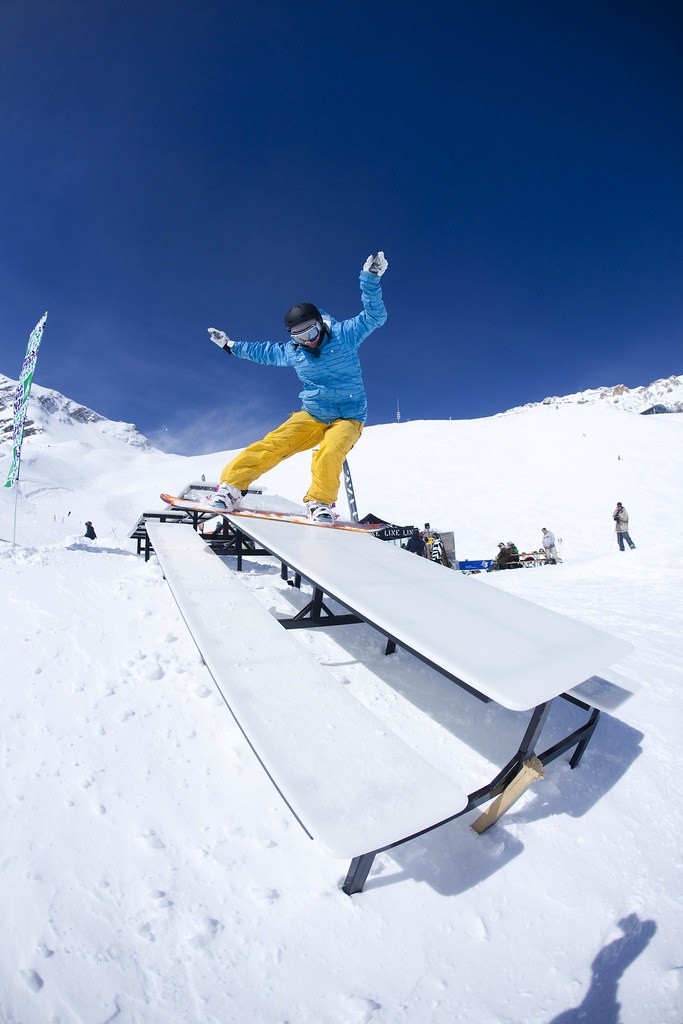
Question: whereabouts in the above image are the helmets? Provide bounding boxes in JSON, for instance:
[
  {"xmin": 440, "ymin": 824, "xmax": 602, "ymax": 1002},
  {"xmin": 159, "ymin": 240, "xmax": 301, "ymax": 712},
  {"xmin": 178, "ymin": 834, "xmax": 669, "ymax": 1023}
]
[
  {"xmin": 539, "ymin": 548, "xmax": 543, "ymax": 551},
  {"xmin": 285, "ymin": 303, "xmax": 323, "ymax": 334},
  {"xmin": 432, "ymin": 533, "xmax": 440, "ymax": 538}
]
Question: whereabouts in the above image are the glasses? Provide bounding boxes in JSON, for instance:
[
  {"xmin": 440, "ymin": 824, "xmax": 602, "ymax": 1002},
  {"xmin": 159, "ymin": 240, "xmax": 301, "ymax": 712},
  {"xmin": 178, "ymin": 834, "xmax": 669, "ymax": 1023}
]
[{"xmin": 291, "ymin": 321, "xmax": 319, "ymax": 343}]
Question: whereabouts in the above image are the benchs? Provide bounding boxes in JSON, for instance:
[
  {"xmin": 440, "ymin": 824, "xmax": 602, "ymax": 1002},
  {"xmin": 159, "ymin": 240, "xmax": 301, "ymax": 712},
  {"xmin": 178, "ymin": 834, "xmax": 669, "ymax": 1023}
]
[
  {"xmin": 505, "ymin": 558, "xmax": 548, "ymax": 568},
  {"xmin": 148, "ymin": 522, "xmax": 637, "ymax": 894}
]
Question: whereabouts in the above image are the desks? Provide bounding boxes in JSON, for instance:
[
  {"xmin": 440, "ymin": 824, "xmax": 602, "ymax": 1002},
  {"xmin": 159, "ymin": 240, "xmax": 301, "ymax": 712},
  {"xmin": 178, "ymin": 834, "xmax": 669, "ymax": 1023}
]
[
  {"xmin": 509, "ymin": 552, "xmax": 544, "ymax": 567},
  {"xmin": 212, "ymin": 494, "xmax": 634, "ymax": 789}
]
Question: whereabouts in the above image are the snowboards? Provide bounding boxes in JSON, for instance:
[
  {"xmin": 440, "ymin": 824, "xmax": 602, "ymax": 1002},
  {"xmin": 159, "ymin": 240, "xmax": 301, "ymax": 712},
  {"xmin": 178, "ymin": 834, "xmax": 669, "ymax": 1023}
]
[{"xmin": 160, "ymin": 491, "xmax": 389, "ymax": 534}]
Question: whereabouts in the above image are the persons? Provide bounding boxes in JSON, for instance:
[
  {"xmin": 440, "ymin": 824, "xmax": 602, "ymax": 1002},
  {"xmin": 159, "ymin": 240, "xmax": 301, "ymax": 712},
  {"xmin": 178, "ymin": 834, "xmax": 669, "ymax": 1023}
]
[
  {"xmin": 405, "ymin": 528, "xmax": 448, "ymax": 566},
  {"xmin": 84, "ymin": 521, "xmax": 96, "ymax": 540},
  {"xmin": 613, "ymin": 502, "xmax": 635, "ymax": 551},
  {"xmin": 207, "ymin": 251, "xmax": 388, "ymax": 523},
  {"xmin": 497, "ymin": 541, "xmax": 519, "ymax": 569},
  {"xmin": 542, "ymin": 528, "xmax": 558, "ymax": 564}
]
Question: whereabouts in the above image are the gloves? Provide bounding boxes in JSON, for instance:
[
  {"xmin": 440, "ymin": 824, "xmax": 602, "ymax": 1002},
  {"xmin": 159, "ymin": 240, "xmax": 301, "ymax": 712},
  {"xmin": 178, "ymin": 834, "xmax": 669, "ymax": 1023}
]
[
  {"xmin": 363, "ymin": 252, "xmax": 388, "ymax": 276},
  {"xmin": 208, "ymin": 328, "xmax": 235, "ymax": 355}
]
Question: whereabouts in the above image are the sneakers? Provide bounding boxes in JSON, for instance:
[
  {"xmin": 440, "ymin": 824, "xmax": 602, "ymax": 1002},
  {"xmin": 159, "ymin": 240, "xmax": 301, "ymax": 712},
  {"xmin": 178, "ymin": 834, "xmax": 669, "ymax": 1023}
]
[
  {"xmin": 305, "ymin": 500, "xmax": 336, "ymax": 525},
  {"xmin": 207, "ymin": 482, "xmax": 242, "ymax": 512}
]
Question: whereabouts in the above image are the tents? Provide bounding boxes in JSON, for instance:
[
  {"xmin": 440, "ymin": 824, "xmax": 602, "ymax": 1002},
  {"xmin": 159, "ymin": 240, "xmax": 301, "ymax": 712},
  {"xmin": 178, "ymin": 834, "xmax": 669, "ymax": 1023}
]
[{"xmin": 359, "ymin": 513, "xmax": 415, "ymax": 546}]
